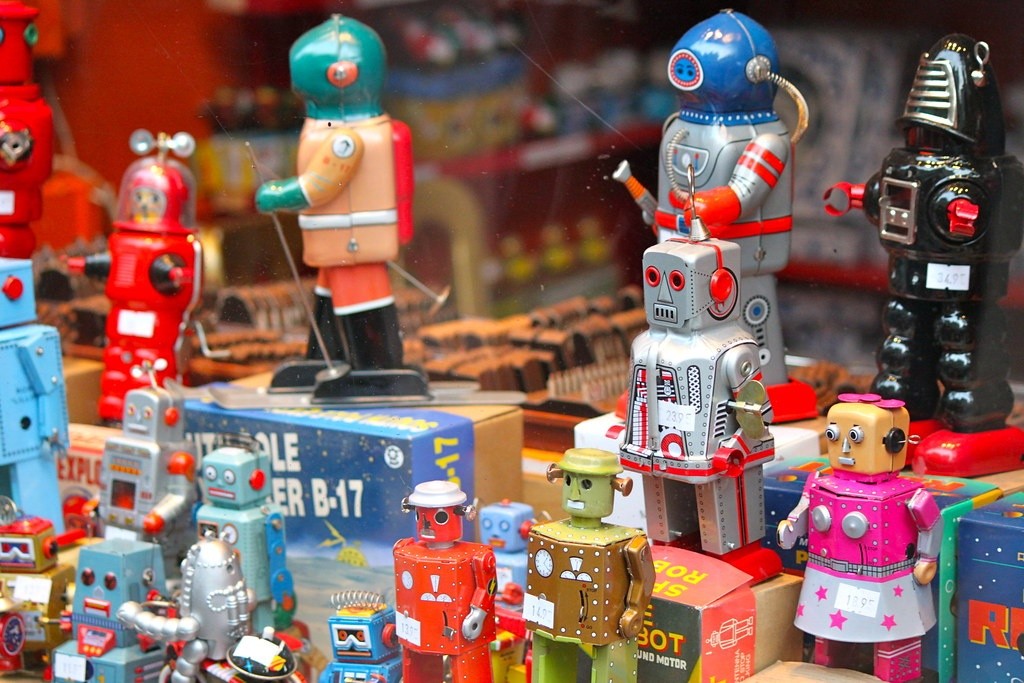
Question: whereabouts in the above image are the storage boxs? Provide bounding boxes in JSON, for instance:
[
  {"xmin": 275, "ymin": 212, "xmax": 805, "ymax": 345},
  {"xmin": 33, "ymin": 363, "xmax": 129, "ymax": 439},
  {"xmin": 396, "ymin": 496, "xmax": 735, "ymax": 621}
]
[{"xmin": 55, "ymin": 356, "xmax": 1024, "ymax": 680}]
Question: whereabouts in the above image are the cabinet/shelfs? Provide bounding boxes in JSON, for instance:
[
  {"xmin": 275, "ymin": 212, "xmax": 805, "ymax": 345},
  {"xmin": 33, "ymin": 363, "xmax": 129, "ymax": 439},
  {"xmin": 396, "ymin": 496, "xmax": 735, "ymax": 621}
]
[{"xmin": 412, "ymin": 124, "xmax": 891, "ymax": 325}]
